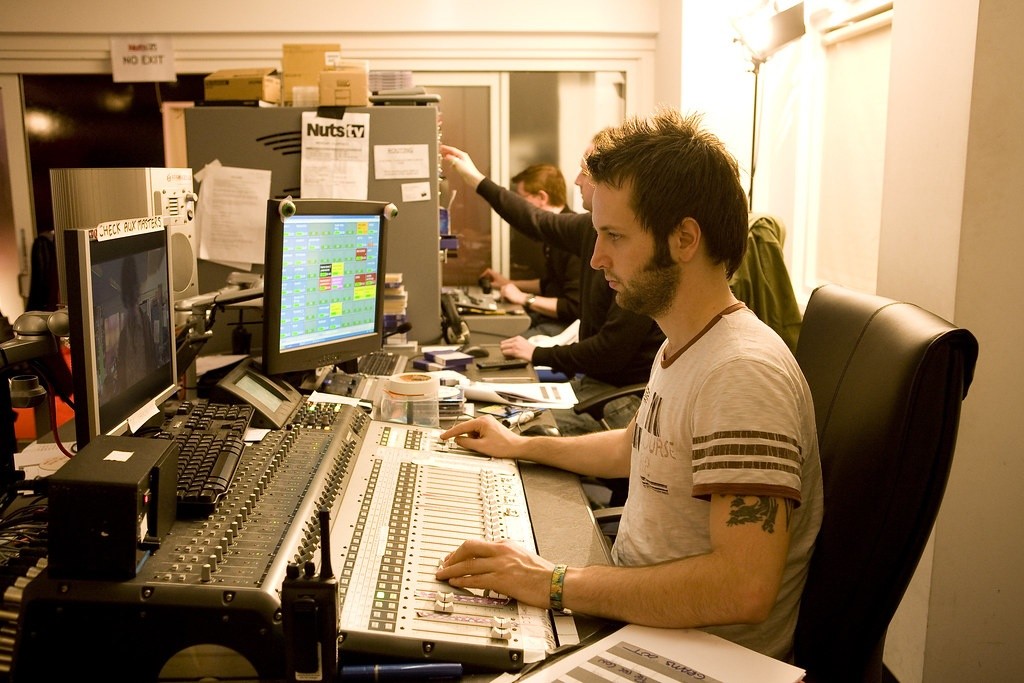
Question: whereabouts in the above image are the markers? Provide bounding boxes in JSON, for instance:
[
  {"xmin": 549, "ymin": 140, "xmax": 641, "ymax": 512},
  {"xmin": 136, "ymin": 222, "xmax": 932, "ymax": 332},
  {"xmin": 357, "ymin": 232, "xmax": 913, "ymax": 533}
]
[
  {"xmin": 338, "ymin": 660, "xmax": 465, "ymax": 683},
  {"xmin": 502, "ymin": 409, "xmax": 543, "ymax": 428}
]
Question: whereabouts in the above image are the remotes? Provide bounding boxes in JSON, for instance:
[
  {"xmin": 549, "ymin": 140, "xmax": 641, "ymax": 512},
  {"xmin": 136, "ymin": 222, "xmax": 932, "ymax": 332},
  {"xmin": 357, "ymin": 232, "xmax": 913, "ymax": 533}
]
[{"xmin": 476, "ymin": 359, "xmax": 529, "ymax": 370}]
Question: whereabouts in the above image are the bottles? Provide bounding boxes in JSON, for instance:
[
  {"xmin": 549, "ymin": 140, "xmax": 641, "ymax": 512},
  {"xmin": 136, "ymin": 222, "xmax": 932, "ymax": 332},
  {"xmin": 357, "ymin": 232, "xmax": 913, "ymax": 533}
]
[{"xmin": 378, "ymin": 375, "xmax": 440, "ymax": 432}]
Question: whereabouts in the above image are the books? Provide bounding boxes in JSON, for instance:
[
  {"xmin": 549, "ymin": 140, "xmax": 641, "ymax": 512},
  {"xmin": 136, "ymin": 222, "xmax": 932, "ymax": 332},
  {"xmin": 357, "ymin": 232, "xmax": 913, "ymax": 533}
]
[{"xmin": 383, "ymin": 273, "xmax": 418, "ymax": 350}]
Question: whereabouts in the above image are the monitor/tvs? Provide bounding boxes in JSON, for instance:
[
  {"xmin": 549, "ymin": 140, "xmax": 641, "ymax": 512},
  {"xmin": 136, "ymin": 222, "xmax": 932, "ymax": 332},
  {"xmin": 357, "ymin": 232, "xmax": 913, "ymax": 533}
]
[
  {"xmin": 263, "ymin": 199, "xmax": 392, "ymax": 395},
  {"xmin": 63, "ymin": 217, "xmax": 178, "ymax": 452}
]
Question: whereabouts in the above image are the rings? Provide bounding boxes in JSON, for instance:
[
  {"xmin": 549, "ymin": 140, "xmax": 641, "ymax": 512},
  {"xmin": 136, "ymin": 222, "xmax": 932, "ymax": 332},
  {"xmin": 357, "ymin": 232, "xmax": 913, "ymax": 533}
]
[{"xmin": 452, "ymin": 158, "xmax": 459, "ymax": 165}]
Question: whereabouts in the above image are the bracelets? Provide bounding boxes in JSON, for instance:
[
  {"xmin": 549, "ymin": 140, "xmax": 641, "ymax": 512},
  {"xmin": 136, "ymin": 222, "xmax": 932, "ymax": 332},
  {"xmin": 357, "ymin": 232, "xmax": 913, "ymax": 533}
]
[{"xmin": 550, "ymin": 565, "xmax": 573, "ymax": 615}]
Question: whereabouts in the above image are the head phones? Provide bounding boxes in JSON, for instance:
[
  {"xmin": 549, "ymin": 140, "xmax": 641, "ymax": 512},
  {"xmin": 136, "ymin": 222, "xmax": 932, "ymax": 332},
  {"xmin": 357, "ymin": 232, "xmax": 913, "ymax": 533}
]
[{"xmin": 441, "ymin": 292, "xmax": 471, "ymax": 345}]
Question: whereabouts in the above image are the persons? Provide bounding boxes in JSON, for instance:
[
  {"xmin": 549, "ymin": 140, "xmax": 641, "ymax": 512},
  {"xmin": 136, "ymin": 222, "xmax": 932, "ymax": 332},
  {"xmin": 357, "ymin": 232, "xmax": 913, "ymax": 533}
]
[
  {"xmin": 481, "ymin": 164, "xmax": 583, "ymax": 340},
  {"xmin": 437, "ymin": 108, "xmax": 824, "ymax": 662},
  {"xmin": 440, "ymin": 133, "xmax": 667, "ymax": 436},
  {"xmin": 118, "ymin": 257, "xmax": 157, "ymax": 391}
]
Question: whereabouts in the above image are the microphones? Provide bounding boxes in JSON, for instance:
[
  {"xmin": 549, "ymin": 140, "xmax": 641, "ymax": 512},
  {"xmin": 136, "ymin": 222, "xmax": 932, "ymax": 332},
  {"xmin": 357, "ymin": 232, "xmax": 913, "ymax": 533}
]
[{"xmin": 382, "ymin": 322, "xmax": 412, "ymax": 339}]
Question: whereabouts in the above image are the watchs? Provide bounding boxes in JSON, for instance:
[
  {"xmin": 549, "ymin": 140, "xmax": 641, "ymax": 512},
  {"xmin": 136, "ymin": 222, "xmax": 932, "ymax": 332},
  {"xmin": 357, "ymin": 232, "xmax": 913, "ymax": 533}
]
[{"xmin": 525, "ymin": 292, "xmax": 535, "ymax": 309}]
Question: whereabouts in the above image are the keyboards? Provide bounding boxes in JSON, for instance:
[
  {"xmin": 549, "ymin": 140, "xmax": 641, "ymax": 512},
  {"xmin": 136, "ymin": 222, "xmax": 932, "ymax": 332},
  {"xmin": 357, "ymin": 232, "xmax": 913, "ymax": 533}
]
[
  {"xmin": 357, "ymin": 352, "xmax": 409, "ymax": 376},
  {"xmin": 152, "ymin": 400, "xmax": 255, "ymax": 512}
]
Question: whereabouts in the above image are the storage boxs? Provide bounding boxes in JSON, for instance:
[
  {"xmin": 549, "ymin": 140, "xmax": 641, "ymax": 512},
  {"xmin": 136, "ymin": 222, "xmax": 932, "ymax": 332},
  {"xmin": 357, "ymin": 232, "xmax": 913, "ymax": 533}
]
[
  {"xmin": 203, "ymin": 66, "xmax": 281, "ymax": 103},
  {"xmin": 277, "ymin": 44, "xmax": 342, "ymax": 100},
  {"xmin": 318, "ymin": 68, "xmax": 370, "ymax": 107}
]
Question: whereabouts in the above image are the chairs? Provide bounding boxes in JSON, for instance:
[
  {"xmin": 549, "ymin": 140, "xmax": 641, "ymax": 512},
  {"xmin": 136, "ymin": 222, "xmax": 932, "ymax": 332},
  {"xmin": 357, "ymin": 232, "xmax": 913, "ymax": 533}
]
[
  {"xmin": 592, "ymin": 283, "xmax": 980, "ymax": 683},
  {"xmin": 573, "ymin": 213, "xmax": 786, "ymax": 431}
]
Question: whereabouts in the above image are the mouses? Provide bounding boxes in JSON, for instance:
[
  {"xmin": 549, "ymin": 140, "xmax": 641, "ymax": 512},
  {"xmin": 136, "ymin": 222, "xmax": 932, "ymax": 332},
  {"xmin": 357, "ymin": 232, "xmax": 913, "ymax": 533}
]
[
  {"xmin": 519, "ymin": 425, "xmax": 563, "ymax": 438},
  {"xmin": 463, "ymin": 346, "xmax": 488, "ymax": 359}
]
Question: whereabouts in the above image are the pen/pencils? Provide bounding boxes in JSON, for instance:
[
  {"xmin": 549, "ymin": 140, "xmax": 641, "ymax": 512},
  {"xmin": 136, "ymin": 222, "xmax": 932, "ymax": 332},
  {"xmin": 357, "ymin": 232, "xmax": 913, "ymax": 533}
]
[{"xmin": 480, "ymin": 377, "xmax": 535, "ymax": 383}]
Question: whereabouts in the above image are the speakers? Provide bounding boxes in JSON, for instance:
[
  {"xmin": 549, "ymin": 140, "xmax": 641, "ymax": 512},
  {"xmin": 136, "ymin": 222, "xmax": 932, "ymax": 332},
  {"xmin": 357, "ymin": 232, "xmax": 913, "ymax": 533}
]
[{"xmin": 51, "ymin": 167, "xmax": 198, "ymax": 327}]
